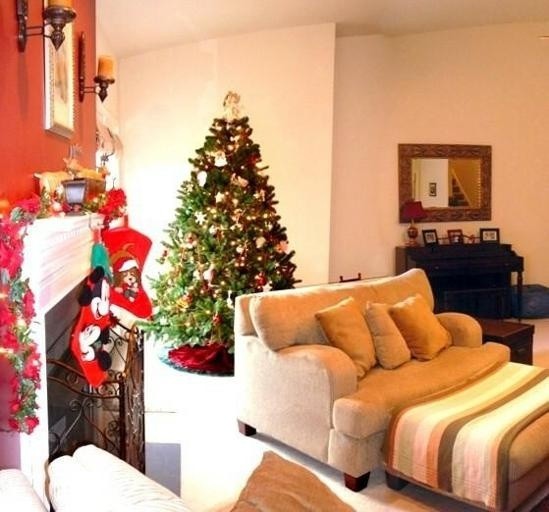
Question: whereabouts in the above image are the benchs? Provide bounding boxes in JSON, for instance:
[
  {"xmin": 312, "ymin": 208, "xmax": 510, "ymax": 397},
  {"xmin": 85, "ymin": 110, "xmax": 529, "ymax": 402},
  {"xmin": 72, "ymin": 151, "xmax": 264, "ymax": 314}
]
[
  {"xmin": 384, "ymin": 361, "xmax": 549, "ymax": 512},
  {"xmin": 441, "ymin": 287, "xmax": 506, "ymax": 320}
]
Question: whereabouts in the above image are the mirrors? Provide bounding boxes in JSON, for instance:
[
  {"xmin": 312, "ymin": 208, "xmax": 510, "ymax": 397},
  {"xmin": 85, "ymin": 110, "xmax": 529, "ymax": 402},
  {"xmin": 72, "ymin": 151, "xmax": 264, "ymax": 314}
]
[{"xmin": 397, "ymin": 143, "xmax": 492, "ymax": 224}]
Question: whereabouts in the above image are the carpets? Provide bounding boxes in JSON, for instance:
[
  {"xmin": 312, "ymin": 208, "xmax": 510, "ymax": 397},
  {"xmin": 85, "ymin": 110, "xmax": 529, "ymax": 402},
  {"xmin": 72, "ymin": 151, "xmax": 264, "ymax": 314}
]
[{"xmin": 510, "ymin": 284, "xmax": 548, "ymax": 318}]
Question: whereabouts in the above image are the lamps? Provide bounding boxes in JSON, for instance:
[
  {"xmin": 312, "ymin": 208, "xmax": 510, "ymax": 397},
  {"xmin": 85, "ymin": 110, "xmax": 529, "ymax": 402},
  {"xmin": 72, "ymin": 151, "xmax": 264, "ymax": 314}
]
[
  {"xmin": 16, "ymin": 0, "xmax": 78, "ymax": 51},
  {"xmin": 401, "ymin": 201, "xmax": 427, "ymax": 248},
  {"xmin": 79, "ymin": 31, "xmax": 117, "ymax": 102}
]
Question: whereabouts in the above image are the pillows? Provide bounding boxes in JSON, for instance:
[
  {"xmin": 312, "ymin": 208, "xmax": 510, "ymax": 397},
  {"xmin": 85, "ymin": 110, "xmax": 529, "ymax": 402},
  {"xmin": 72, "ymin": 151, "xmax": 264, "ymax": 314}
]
[
  {"xmin": 388, "ymin": 293, "xmax": 452, "ymax": 361},
  {"xmin": 362, "ymin": 301, "xmax": 410, "ymax": 370},
  {"xmin": 315, "ymin": 297, "xmax": 376, "ymax": 377}
]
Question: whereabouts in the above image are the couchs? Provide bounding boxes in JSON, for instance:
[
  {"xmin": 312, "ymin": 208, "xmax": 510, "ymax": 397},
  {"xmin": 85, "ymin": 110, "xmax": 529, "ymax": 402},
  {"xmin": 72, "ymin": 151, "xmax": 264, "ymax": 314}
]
[{"xmin": 235, "ymin": 268, "xmax": 510, "ymax": 493}]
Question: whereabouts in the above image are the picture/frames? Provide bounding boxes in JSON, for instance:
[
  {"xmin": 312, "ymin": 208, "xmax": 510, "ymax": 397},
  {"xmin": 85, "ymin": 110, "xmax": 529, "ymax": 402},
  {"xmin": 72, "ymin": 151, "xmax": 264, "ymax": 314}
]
[
  {"xmin": 448, "ymin": 230, "xmax": 462, "ymax": 245},
  {"xmin": 428, "ymin": 183, "xmax": 436, "ymax": 196},
  {"xmin": 43, "ymin": 0, "xmax": 77, "ymax": 141},
  {"xmin": 480, "ymin": 229, "xmax": 498, "ymax": 242},
  {"xmin": 423, "ymin": 230, "xmax": 437, "ymax": 246}
]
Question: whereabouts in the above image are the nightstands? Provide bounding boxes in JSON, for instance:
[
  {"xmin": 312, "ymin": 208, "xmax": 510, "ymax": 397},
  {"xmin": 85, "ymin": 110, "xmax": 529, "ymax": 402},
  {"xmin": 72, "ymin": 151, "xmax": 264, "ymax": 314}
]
[{"xmin": 482, "ymin": 322, "xmax": 534, "ymax": 366}]
